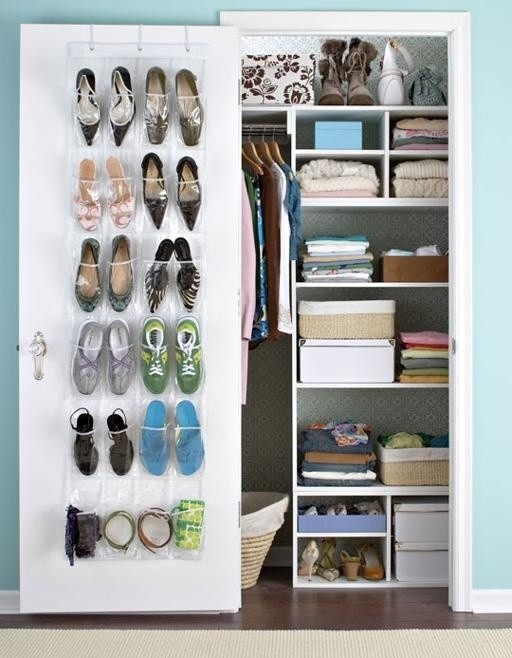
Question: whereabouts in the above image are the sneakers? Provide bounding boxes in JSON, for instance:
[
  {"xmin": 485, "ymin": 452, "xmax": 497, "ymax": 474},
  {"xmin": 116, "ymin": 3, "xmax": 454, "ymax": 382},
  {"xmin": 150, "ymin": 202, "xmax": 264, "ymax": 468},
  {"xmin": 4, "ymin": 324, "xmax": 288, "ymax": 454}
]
[{"xmin": 72, "ymin": 319, "xmax": 201, "ymax": 389}]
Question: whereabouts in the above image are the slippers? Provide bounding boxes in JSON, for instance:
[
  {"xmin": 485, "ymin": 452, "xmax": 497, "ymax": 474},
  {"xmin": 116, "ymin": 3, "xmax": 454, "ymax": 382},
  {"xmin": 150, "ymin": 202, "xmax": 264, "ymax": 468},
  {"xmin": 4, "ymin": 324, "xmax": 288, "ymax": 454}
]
[
  {"xmin": 174, "ymin": 399, "xmax": 204, "ymax": 475},
  {"xmin": 139, "ymin": 400, "xmax": 168, "ymax": 476}
]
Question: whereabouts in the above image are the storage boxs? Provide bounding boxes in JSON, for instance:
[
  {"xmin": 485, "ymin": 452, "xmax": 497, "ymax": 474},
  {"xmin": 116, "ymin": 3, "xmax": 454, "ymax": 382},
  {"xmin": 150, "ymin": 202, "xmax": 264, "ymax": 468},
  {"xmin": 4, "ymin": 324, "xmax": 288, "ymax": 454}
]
[
  {"xmin": 314, "ymin": 119, "xmax": 364, "ymax": 150},
  {"xmin": 296, "ymin": 336, "xmax": 398, "ymax": 385},
  {"xmin": 390, "ymin": 496, "xmax": 451, "ymax": 582}
]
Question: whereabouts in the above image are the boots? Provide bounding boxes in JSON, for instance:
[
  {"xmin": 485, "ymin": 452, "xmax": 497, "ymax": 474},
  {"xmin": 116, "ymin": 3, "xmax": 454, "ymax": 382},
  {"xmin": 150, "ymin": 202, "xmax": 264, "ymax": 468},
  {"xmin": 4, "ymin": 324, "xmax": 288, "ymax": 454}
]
[
  {"xmin": 317, "ymin": 39, "xmax": 346, "ymax": 105},
  {"xmin": 344, "ymin": 38, "xmax": 378, "ymax": 105}
]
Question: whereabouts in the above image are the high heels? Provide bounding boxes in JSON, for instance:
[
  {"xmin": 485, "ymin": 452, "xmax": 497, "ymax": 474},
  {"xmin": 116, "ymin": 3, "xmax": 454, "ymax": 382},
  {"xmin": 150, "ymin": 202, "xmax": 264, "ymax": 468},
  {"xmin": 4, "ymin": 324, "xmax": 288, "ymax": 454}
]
[{"xmin": 299, "ymin": 536, "xmax": 384, "ymax": 581}]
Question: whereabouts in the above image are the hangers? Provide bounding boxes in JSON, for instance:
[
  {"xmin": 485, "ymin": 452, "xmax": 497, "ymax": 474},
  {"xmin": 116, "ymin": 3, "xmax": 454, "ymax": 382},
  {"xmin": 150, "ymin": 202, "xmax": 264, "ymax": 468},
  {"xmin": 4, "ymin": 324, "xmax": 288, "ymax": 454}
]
[{"xmin": 240, "ymin": 124, "xmax": 294, "ymax": 182}]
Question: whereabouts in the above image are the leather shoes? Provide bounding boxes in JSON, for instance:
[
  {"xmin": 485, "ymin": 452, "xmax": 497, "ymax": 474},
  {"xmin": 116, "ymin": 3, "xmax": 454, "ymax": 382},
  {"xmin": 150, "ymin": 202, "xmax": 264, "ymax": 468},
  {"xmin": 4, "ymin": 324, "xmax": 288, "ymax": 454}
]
[{"xmin": 73, "ymin": 69, "xmax": 204, "ymax": 307}]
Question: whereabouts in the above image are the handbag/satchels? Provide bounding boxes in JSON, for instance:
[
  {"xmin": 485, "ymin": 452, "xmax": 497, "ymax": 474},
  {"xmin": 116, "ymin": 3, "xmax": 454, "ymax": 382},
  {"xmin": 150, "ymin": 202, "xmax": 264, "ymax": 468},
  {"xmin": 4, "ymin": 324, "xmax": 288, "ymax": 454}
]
[
  {"xmin": 376, "ymin": 38, "xmax": 414, "ymax": 106},
  {"xmin": 170, "ymin": 500, "xmax": 204, "ymax": 550},
  {"xmin": 406, "ymin": 65, "xmax": 448, "ymax": 107}
]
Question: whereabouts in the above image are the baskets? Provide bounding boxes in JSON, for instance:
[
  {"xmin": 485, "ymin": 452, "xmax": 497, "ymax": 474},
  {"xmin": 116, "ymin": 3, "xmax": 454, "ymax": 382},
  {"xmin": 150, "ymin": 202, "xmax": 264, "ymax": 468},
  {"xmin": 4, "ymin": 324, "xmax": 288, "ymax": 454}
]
[
  {"xmin": 298, "ymin": 300, "xmax": 396, "ymax": 340},
  {"xmin": 241, "ymin": 492, "xmax": 289, "ymax": 590},
  {"xmin": 376, "ymin": 439, "xmax": 450, "ymax": 485}
]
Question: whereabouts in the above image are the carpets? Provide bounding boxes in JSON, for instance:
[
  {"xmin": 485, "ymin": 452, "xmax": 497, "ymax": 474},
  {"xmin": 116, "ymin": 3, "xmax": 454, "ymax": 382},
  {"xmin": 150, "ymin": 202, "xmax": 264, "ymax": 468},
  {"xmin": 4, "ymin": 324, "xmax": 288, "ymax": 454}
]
[{"xmin": 0, "ymin": 623, "xmax": 510, "ymax": 657}]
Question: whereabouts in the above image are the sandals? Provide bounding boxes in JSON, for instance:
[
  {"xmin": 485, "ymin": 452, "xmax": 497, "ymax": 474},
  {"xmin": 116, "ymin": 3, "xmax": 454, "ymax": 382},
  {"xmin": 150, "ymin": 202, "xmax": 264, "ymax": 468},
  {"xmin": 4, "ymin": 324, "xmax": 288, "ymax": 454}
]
[
  {"xmin": 107, "ymin": 408, "xmax": 134, "ymax": 476},
  {"xmin": 70, "ymin": 408, "xmax": 99, "ymax": 475}
]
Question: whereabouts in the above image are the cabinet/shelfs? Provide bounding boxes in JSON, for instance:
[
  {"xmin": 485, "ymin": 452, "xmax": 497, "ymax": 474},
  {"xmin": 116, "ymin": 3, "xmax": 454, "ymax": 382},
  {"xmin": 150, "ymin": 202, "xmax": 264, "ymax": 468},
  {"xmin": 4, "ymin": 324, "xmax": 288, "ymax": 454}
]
[{"xmin": 235, "ymin": 34, "xmax": 455, "ymax": 588}]
